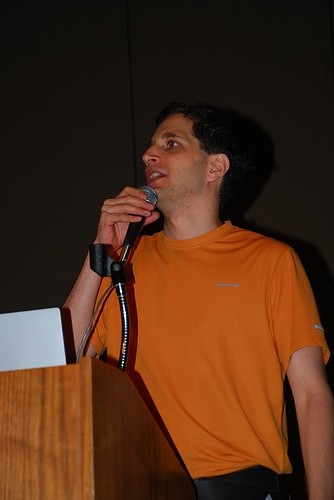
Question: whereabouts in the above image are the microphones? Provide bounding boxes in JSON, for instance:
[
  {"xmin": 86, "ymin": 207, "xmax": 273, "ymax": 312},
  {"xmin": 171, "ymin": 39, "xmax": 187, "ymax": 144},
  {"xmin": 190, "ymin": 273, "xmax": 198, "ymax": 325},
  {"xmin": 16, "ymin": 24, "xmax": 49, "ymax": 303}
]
[{"xmin": 119, "ymin": 186, "xmax": 158, "ymax": 267}]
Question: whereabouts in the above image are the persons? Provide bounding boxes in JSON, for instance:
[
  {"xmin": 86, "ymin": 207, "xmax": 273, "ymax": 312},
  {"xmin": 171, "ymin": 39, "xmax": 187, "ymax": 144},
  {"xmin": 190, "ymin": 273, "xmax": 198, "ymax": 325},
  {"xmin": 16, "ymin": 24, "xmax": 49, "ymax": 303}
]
[{"xmin": 58, "ymin": 98, "xmax": 334, "ymax": 500}]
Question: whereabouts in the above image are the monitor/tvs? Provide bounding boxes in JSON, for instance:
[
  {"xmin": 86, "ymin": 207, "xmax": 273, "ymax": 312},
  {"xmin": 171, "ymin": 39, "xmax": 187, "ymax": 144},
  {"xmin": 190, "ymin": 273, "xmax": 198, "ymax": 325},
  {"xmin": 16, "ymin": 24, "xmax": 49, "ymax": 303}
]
[{"xmin": 1, "ymin": 307, "xmax": 67, "ymax": 370}]
